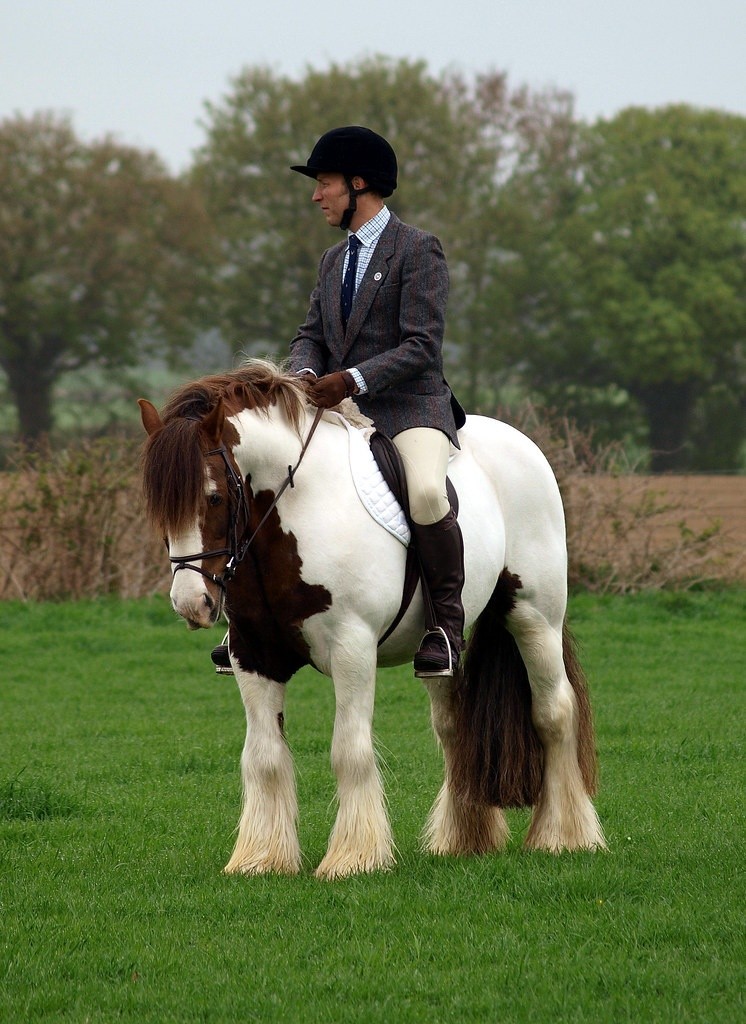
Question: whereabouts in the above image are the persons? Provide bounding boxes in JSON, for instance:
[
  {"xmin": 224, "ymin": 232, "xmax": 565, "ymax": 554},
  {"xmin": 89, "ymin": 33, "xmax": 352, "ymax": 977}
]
[{"xmin": 211, "ymin": 127, "xmax": 467, "ymax": 669}]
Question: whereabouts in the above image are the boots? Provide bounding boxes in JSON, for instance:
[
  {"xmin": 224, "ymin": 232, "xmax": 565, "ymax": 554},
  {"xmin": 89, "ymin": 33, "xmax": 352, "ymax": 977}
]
[
  {"xmin": 410, "ymin": 507, "xmax": 467, "ymax": 673},
  {"xmin": 210, "ymin": 645, "xmax": 231, "ymax": 668}
]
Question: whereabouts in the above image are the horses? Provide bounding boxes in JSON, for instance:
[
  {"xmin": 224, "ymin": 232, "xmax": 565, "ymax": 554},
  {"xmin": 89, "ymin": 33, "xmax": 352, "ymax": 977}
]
[{"xmin": 138, "ymin": 354, "xmax": 609, "ymax": 879}]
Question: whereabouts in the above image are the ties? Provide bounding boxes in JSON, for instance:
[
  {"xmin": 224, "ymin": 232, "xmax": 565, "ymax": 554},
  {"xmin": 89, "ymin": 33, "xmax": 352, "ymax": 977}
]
[{"xmin": 340, "ymin": 236, "xmax": 362, "ymax": 341}]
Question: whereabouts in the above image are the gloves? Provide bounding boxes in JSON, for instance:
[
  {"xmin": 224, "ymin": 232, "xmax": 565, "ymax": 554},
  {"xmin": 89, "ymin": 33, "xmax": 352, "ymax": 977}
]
[{"xmin": 296, "ymin": 370, "xmax": 360, "ymax": 408}]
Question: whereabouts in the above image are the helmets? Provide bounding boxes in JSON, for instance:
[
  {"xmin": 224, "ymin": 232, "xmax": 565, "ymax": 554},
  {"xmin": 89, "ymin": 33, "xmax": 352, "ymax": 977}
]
[{"xmin": 290, "ymin": 126, "xmax": 398, "ymax": 198}]
